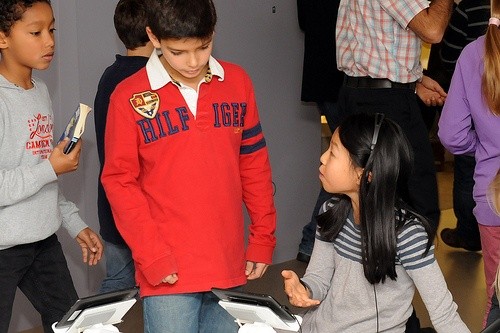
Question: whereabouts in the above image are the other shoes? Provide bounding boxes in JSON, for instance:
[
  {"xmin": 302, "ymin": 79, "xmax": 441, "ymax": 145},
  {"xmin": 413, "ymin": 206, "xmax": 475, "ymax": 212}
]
[
  {"xmin": 296, "ymin": 247, "xmax": 312, "ymax": 262},
  {"xmin": 440, "ymin": 227, "xmax": 482, "ymax": 251}
]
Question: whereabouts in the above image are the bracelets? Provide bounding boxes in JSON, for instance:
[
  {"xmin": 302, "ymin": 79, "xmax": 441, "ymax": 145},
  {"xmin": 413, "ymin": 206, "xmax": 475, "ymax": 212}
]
[{"xmin": 414, "ymin": 72, "xmax": 424, "ymax": 94}]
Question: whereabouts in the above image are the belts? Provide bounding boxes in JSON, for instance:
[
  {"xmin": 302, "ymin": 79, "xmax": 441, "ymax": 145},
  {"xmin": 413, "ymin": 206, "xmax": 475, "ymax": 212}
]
[{"xmin": 341, "ymin": 76, "xmax": 414, "ymax": 89}]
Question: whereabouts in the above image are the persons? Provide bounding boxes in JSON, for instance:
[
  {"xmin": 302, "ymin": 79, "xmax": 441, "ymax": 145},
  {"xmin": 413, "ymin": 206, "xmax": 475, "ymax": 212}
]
[
  {"xmin": 336, "ymin": 0, "xmax": 457, "ymax": 250},
  {"xmin": 437, "ymin": 0, "xmax": 500, "ymax": 333},
  {"xmin": 282, "ymin": 113, "xmax": 474, "ymax": 333},
  {"xmin": 295, "ymin": 0, "xmax": 347, "ymax": 263},
  {"xmin": 428, "ymin": 0, "xmax": 493, "ymax": 252},
  {"xmin": 0, "ymin": 0, "xmax": 104, "ymax": 333},
  {"xmin": 94, "ymin": 0, "xmax": 155, "ymax": 295},
  {"xmin": 100, "ymin": 0, "xmax": 277, "ymax": 333}
]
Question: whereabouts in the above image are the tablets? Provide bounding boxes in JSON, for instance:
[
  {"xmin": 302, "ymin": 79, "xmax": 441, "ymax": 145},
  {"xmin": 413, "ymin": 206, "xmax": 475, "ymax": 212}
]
[
  {"xmin": 55, "ymin": 285, "xmax": 140, "ymax": 329},
  {"xmin": 211, "ymin": 288, "xmax": 296, "ymax": 323}
]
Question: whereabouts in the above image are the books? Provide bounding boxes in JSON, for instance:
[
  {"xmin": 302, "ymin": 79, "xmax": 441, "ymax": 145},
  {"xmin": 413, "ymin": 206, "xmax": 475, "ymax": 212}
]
[{"xmin": 56, "ymin": 102, "xmax": 93, "ymax": 154}]
